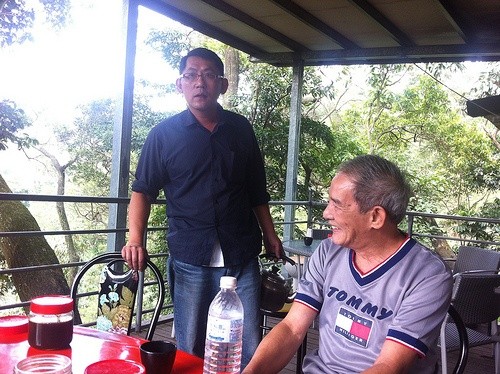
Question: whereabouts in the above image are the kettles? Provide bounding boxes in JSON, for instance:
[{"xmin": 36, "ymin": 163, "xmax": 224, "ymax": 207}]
[{"xmin": 259, "ymin": 253, "xmax": 297, "ymax": 313}]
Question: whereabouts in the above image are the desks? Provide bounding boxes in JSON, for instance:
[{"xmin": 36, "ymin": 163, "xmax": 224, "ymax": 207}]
[
  {"xmin": 282, "ymin": 238, "xmax": 325, "ymax": 328},
  {"xmin": 0, "ymin": 315, "xmax": 229, "ymax": 374}
]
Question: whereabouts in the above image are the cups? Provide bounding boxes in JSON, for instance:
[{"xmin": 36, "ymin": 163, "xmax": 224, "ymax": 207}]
[
  {"xmin": 139, "ymin": 339, "xmax": 177, "ymax": 374},
  {"xmin": 14, "ymin": 353, "xmax": 74, "ymax": 374},
  {"xmin": 84, "ymin": 358, "xmax": 146, "ymax": 374},
  {"xmin": 304, "ymin": 237, "xmax": 313, "ymax": 246}
]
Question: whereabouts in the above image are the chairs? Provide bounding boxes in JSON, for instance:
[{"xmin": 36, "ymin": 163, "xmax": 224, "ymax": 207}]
[
  {"xmin": 301, "ymin": 228, "xmax": 332, "ymax": 280},
  {"xmin": 257, "ymin": 252, "xmax": 308, "ymax": 374},
  {"xmin": 71, "ymin": 252, "xmax": 165, "ymax": 340},
  {"xmin": 429, "ymin": 245, "xmax": 500, "ymax": 374}
]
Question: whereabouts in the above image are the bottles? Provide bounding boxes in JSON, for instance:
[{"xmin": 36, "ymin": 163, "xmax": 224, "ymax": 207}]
[
  {"xmin": 25, "ymin": 293, "xmax": 74, "ymax": 361},
  {"xmin": 203, "ymin": 276, "xmax": 244, "ymax": 374},
  {"xmin": 0, "ymin": 315, "xmax": 31, "ymax": 374}
]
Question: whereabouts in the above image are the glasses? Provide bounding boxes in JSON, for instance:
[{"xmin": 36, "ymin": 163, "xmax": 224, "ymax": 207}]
[{"xmin": 180, "ymin": 72, "xmax": 224, "ymax": 81}]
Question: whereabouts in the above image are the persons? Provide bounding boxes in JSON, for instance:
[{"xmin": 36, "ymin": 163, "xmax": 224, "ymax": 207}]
[
  {"xmin": 122, "ymin": 48, "xmax": 287, "ymax": 374},
  {"xmin": 240, "ymin": 155, "xmax": 453, "ymax": 374}
]
